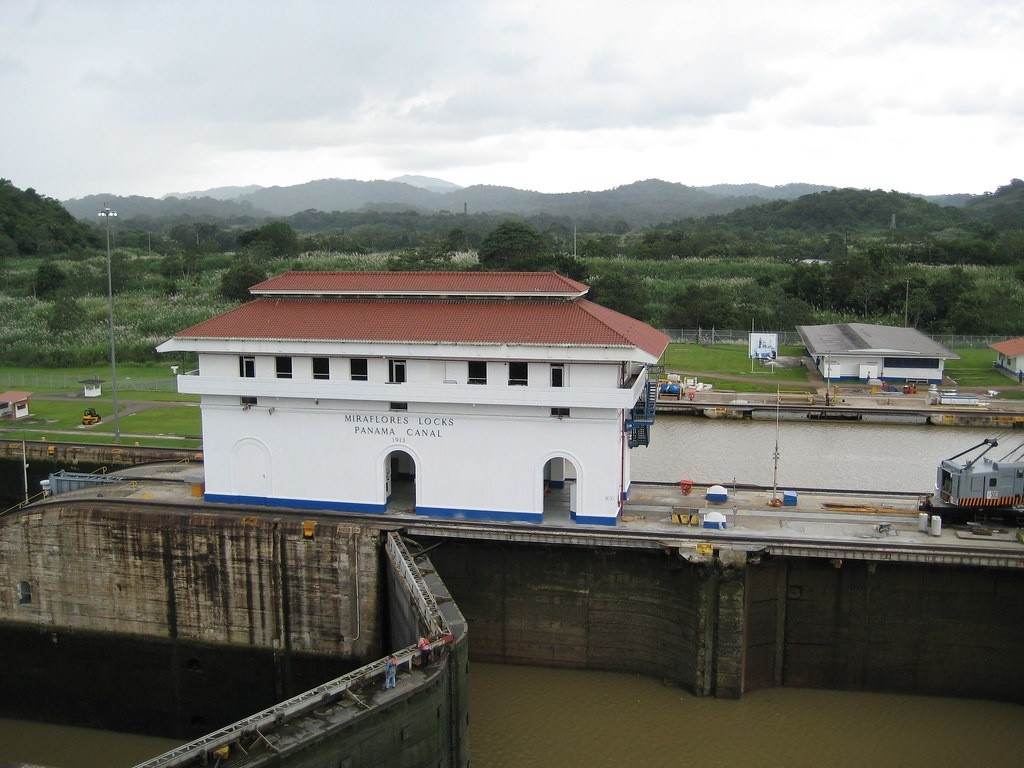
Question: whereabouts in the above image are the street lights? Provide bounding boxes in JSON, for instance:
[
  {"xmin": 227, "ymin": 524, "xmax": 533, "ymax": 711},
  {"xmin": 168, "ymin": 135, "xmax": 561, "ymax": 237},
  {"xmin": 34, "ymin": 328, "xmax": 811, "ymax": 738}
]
[{"xmin": 97, "ymin": 204, "xmax": 121, "ymax": 445}]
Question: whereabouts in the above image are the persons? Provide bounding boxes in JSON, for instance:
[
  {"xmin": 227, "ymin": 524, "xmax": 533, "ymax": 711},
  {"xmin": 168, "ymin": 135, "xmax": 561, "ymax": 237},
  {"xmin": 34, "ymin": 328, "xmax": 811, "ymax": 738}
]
[
  {"xmin": 418, "ymin": 634, "xmax": 430, "ymax": 671},
  {"xmin": 384, "ymin": 654, "xmax": 396, "ymax": 690},
  {"xmin": 883, "ymin": 383, "xmax": 899, "ymax": 392},
  {"xmin": 1019, "ymin": 370, "xmax": 1022, "ymax": 383},
  {"xmin": 866, "ymin": 372, "xmax": 870, "ymax": 383}
]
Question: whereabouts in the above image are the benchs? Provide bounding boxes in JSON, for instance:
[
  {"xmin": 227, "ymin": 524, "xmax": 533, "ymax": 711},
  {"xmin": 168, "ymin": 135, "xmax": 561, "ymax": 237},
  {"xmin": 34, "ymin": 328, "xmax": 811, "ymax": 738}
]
[
  {"xmin": 703, "ymin": 512, "xmax": 727, "ymax": 529},
  {"xmin": 706, "ymin": 485, "xmax": 728, "ymax": 501}
]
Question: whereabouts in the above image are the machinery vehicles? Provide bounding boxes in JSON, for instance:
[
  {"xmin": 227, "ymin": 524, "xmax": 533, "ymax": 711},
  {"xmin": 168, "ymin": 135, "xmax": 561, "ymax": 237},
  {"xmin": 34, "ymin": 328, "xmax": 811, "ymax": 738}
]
[
  {"xmin": 918, "ymin": 427, "xmax": 1024, "ymax": 525},
  {"xmin": 82, "ymin": 408, "xmax": 101, "ymax": 425}
]
